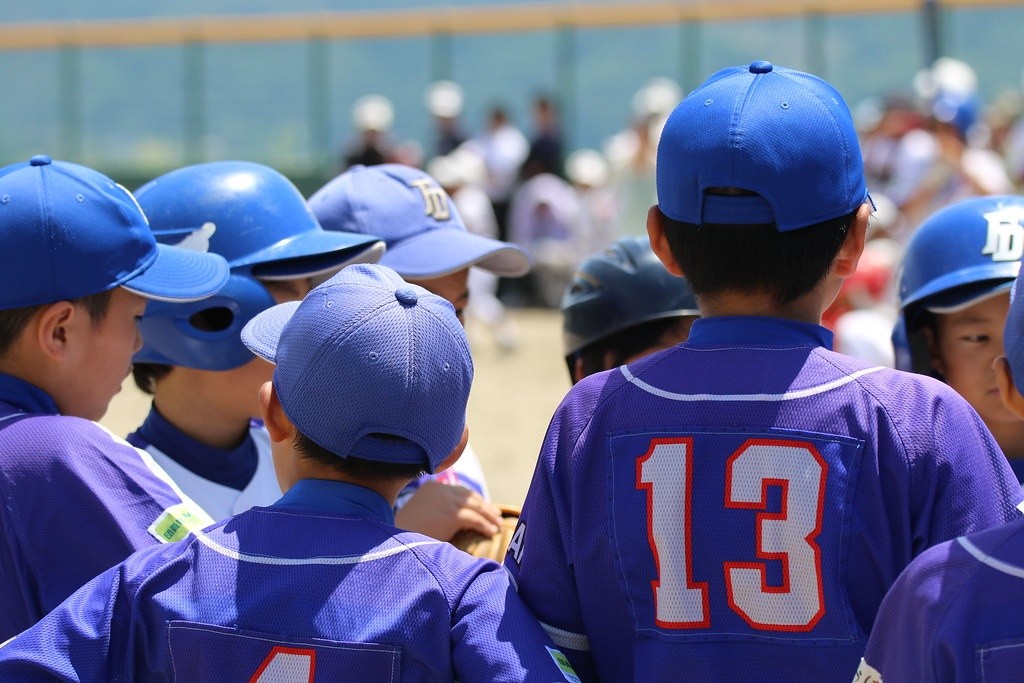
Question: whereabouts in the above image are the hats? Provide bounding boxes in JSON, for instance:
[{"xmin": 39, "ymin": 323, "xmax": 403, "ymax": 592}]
[
  {"xmin": 353, "ymin": 94, "xmax": 393, "ymax": 132},
  {"xmin": 923, "ymin": 279, "xmax": 1016, "ymax": 314},
  {"xmin": 307, "ymin": 162, "xmax": 533, "ymax": 280},
  {"xmin": 0, "ymin": 154, "xmax": 229, "ymax": 309},
  {"xmin": 241, "ymin": 264, "xmax": 473, "ymax": 476},
  {"xmin": 423, "ymin": 80, "xmax": 464, "ymax": 118},
  {"xmin": 656, "ymin": 60, "xmax": 878, "ymax": 230},
  {"xmin": 1003, "ymin": 262, "xmax": 1024, "ymax": 396},
  {"xmin": 252, "ymin": 240, "xmax": 386, "ymax": 288}
]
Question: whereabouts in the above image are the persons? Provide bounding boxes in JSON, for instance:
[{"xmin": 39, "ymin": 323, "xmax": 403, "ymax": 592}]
[
  {"xmin": 0, "ymin": 263, "xmax": 580, "ymax": 682},
  {"xmin": 895, "ymin": 194, "xmax": 1024, "ymax": 485},
  {"xmin": 337, "ymin": 49, "xmax": 1024, "ymax": 369},
  {"xmin": 560, "ymin": 233, "xmax": 700, "ymax": 386},
  {"xmin": 507, "ymin": 63, "xmax": 1024, "ymax": 682},
  {"xmin": 0, "ymin": 155, "xmax": 534, "ymax": 646},
  {"xmin": 848, "ymin": 260, "xmax": 1024, "ymax": 683}
]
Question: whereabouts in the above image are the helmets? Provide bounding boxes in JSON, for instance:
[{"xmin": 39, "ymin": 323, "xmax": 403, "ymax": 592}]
[
  {"xmin": 132, "ymin": 161, "xmax": 384, "ymax": 372},
  {"xmin": 892, "ymin": 194, "xmax": 1024, "ymax": 375},
  {"xmin": 560, "ymin": 235, "xmax": 702, "ymax": 357}
]
